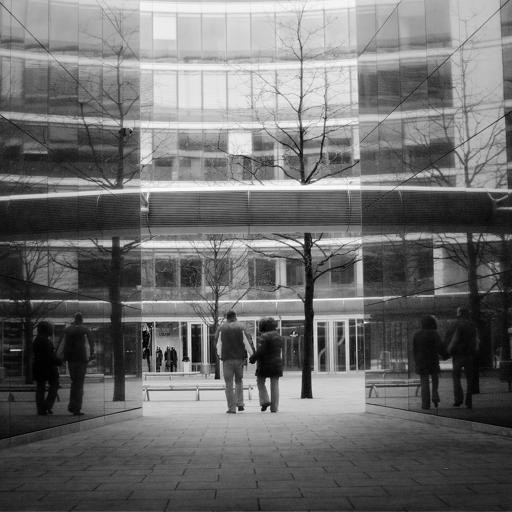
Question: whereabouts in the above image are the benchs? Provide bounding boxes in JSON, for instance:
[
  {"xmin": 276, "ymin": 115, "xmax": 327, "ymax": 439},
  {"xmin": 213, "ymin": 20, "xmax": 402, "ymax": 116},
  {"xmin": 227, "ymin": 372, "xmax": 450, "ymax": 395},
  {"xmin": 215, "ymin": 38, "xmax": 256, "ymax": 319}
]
[
  {"xmin": 142, "ymin": 371, "xmax": 201, "ymax": 381},
  {"xmin": 365, "ymin": 382, "xmax": 422, "ymax": 398},
  {"xmin": 143, "ymin": 384, "xmax": 253, "ymax": 402}
]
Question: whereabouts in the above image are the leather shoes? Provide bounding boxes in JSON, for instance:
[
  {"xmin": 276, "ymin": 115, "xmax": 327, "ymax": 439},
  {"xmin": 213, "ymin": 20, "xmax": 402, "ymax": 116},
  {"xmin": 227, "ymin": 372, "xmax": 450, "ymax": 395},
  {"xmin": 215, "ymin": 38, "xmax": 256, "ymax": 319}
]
[
  {"xmin": 238, "ymin": 406, "xmax": 244, "ymax": 411},
  {"xmin": 432, "ymin": 400, "xmax": 438, "ymax": 408},
  {"xmin": 226, "ymin": 410, "xmax": 236, "ymax": 413},
  {"xmin": 37, "ymin": 405, "xmax": 53, "ymax": 415},
  {"xmin": 67, "ymin": 406, "xmax": 85, "ymax": 415},
  {"xmin": 453, "ymin": 401, "xmax": 463, "ymax": 407},
  {"xmin": 261, "ymin": 402, "xmax": 271, "ymax": 411}
]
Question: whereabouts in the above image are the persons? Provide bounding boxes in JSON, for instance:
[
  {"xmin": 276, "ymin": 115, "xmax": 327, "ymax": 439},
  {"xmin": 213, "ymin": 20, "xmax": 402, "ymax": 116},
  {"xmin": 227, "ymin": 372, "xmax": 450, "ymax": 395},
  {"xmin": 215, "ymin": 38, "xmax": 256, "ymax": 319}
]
[
  {"xmin": 412, "ymin": 306, "xmax": 479, "ymax": 410},
  {"xmin": 498, "ymin": 322, "xmax": 512, "ymax": 392},
  {"xmin": 156, "ymin": 346, "xmax": 177, "ymax": 372},
  {"xmin": 142, "ymin": 322, "xmax": 151, "ymax": 371},
  {"xmin": 28, "ymin": 312, "xmax": 94, "ymax": 416},
  {"xmin": 213, "ymin": 310, "xmax": 285, "ymax": 414}
]
